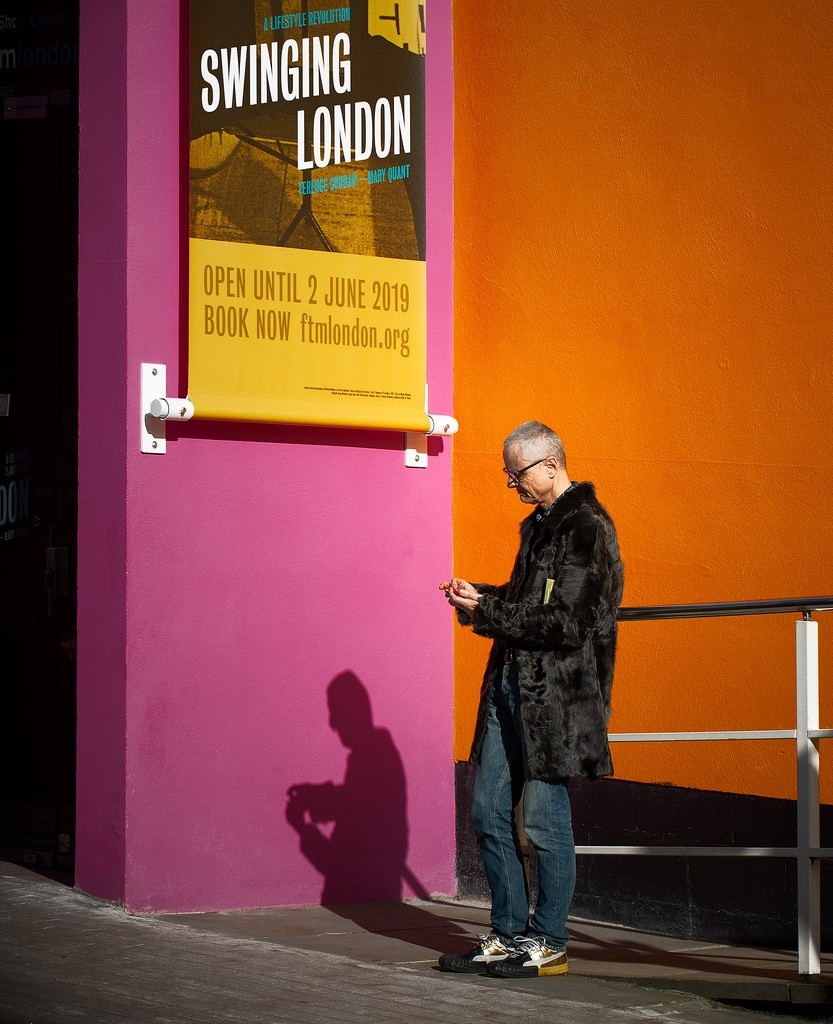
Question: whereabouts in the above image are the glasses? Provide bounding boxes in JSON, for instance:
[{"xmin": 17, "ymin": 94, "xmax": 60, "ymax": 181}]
[{"xmin": 502, "ymin": 458, "xmax": 558, "ymax": 483}]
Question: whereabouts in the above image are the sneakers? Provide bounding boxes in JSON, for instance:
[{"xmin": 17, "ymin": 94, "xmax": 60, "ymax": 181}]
[
  {"xmin": 438, "ymin": 930, "xmax": 515, "ymax": 973},
  {"xmin": 478, "ymin": 936, "xmax": 569, "ymax": 978}
]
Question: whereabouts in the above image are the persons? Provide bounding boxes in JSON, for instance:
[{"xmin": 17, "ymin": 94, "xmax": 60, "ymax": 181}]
[{"xmin": 438, "ymin": 420, "xmax": 625, "ymax": 978}]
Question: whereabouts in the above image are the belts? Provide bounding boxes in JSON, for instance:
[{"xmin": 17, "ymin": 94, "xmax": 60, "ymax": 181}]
[{"xmin": 502, "ymin": 649, "xmax": 517, "ymax": 665}]
